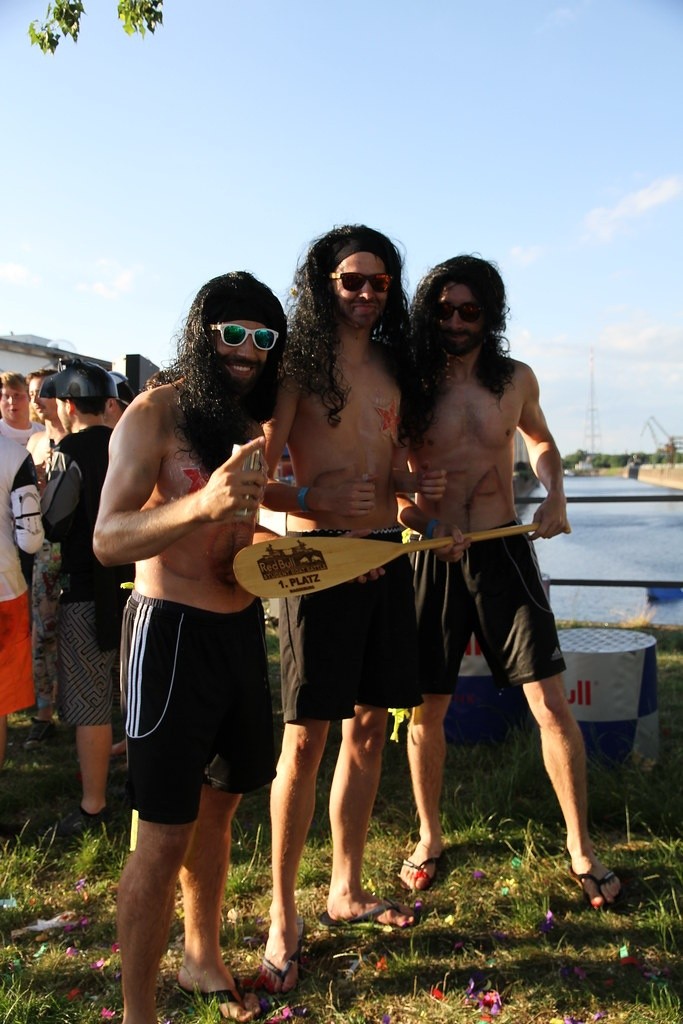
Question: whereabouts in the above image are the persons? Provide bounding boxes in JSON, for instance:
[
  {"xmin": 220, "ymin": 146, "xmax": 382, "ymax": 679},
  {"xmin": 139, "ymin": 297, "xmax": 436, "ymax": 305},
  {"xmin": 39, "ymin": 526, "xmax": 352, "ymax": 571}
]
[
  {"xmin": 0, "ymin": 358, "xmax": 190, "ymax": 843},
  {"xmin": 395, "ymin": 255, "xmax": 623, "ymax": 908},
  {"xmin": 95, "ymin": 271, "xmax": 388, "ymax": 1024},
  {"xmin": 263, "ymin": 226, "xmax": 449, "ymax": 992}
]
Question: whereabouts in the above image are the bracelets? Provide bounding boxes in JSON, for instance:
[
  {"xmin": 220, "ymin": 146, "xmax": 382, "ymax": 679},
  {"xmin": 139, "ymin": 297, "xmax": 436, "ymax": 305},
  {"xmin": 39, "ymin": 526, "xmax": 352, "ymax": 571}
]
[
  {"xmin": 297, "ymin": 487, "xmax": 311, "ymax": 512},
  {"xmin": 426, "ymin": 518, "xmax": 439, "ymax": 538}
]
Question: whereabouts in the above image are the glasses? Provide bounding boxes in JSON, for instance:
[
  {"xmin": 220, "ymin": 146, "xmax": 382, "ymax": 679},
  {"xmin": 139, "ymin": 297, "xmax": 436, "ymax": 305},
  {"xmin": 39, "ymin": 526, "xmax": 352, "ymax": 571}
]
[
  {"xmin": 439, "ymin": 300, "xmax": 483, "ymax": 323},
  {"xmin": 329, "ymin": 272, "xmax": 393, "ymax": 293},
  {"xmin": 210, "ymin": 323, "xmax": 279, "ymax": 350}
]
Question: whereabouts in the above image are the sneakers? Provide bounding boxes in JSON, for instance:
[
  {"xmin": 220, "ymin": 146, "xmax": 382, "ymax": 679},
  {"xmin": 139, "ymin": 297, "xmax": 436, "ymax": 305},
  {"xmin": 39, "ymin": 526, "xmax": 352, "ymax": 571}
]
[
  {"xmin": 24, "ymin": 717, "xmax": 58, "ymax": 750},
  {"xmin": 38, "ymin": 807, "xmax": 112, "ymax": 840}
]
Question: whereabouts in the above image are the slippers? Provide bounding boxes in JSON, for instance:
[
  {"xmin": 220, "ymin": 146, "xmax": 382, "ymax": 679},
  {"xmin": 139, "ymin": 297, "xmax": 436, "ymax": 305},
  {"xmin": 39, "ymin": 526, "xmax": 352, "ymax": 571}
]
[
  {"xmin": 400, "ymin": 851, "xmax": 440, "ymax": 891},
  {"xmin": 179, "ymin": 973, "xmax": 264, "ymax": 1022},
  {"xmin": 569, "ymin": 864, "xmax": 622, "ymax": 903},
  {"xmin": 260, "ymin": 915, "xmax": 304, "ymax": 995},
  {"xmin": 320, "ymin": 896, "xmax": 418, "ymax": 928}
]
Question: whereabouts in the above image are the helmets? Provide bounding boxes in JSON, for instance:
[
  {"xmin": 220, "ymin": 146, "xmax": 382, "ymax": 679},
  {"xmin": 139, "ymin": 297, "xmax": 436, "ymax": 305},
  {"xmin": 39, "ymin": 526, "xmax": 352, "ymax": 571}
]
[
  {"xmin": 107, "ymin": 371, "xmax": 129, "ymax": 385},
  {"xmin": 38, "ymin": 360, "xmax": 118, "ymax": 398}
]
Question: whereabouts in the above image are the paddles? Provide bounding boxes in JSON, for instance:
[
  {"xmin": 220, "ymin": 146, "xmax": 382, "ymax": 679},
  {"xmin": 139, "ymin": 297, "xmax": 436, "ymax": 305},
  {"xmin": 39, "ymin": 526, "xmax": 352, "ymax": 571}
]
[{"xmin": 232, "ymin": 518, "xmax": 571, "ymax": 598}]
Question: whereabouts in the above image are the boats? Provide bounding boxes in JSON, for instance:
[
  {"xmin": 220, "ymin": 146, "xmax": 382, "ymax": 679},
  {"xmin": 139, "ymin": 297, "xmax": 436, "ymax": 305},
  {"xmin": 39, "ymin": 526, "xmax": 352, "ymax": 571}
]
[
  {"xmin": 511, "ymin": 431, "xmax": 540, "ymax": 506},
  {"xmin": 564, "ymin": 470, "xmax": 575, "ymax": 477}
]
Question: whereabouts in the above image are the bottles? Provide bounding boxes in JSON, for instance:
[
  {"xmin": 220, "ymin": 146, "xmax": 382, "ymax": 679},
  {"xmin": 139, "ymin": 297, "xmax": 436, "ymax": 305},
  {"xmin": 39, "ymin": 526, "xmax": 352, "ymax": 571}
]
[{"xmin": 45, "ymin": 439, "xmax": 55, "ymax": 484}]
